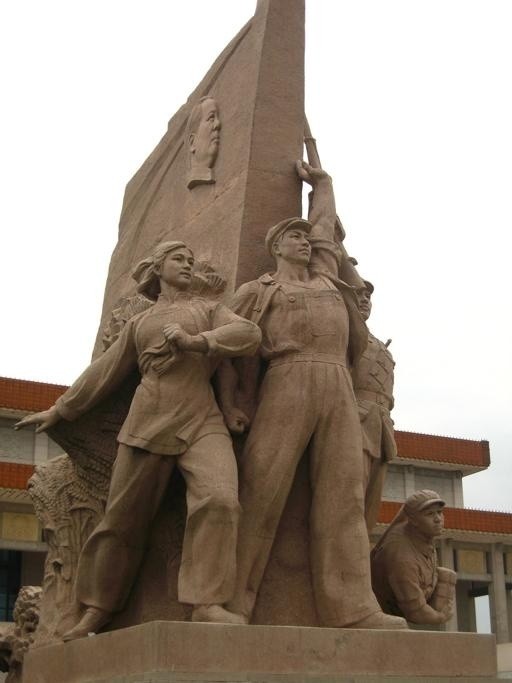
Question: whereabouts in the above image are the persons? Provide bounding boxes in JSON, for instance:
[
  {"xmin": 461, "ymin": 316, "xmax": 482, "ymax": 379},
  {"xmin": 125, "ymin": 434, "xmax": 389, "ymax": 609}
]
[
  {"xmin": 338, "ymin": 255, "xmax": 400, "ymax": 534},
  {"xmin": 211, "ymin": 158, "xmax": 410, "ymax": 628},
  {"xmin": 369, "ymin": 488, "xmax": 458, "ymax": 626},
  {"xmin": 185, "ymin": 94, "xmax": 222, "ymax": 185},
  {"xmin": 13, "ymin": 238, "xmax": 264, "ymax": 644}
]
